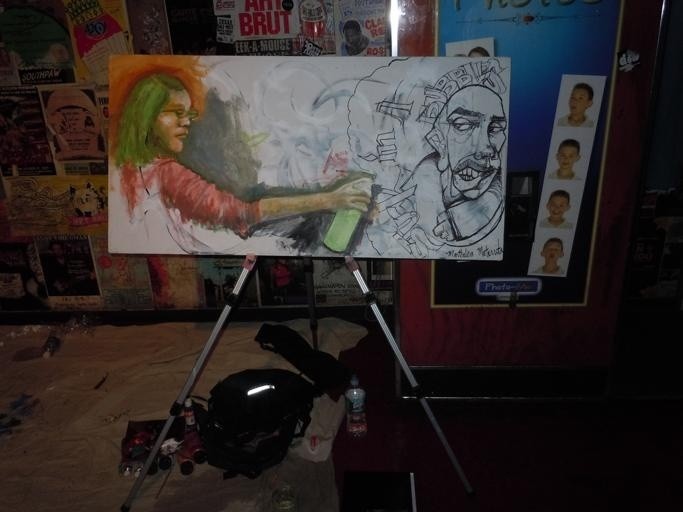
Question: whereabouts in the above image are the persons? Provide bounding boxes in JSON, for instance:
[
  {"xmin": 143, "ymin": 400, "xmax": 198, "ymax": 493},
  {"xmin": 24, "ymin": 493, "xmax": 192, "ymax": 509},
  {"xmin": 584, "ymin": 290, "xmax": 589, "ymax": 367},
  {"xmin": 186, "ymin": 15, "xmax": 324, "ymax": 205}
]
[
  {"xmin": 109, "ymin": 74, "xmax": 370, "ymax": 257},
  {"xmin": 340, "ymin": 19, "xmax": 370, "ymax": 56},
  {"xmin": 45, "ymin": 244, "xmax": 73, "ymax": 294},
  {"xmin": 558, "ymin": 83, "xmax": 594, "ymax": 128},
  {"xmin": 468, "ymin": 45, "xmax": 491, "ymax": 57},
  {"xmin": 538, "ymin": 189, "xmax": 575, "ymax": 229},
  {"xmin": 533, "ymin": 237, "xmax": 566, "ymax": 277},
  {"xmin": 547, "ymin": 138, "xmax": 581, "ymax": 181}
]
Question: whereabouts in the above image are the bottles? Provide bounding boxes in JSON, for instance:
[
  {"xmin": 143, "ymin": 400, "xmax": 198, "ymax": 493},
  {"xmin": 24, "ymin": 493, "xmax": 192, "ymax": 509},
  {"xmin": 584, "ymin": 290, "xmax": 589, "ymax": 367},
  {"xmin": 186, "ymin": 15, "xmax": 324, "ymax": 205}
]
[
  {"xmin": 344, "ymin": 374, "xmax": 369, "ymax": 441},
  {"xmin": 323, "ymin": 178, "xmax": 373, "ymax": 253},
  {"xmin": 272, "ymin": 480, "xmax": 297, "ymax": 512}
]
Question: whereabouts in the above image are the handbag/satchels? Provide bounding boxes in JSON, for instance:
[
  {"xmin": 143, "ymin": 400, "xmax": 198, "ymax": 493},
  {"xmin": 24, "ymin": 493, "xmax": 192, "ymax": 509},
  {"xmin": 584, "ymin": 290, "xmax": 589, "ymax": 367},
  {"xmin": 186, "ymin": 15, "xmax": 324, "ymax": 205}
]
[{"xmin": 208, "ymin": 370, "xmax": 313, "ymax": 444}]
